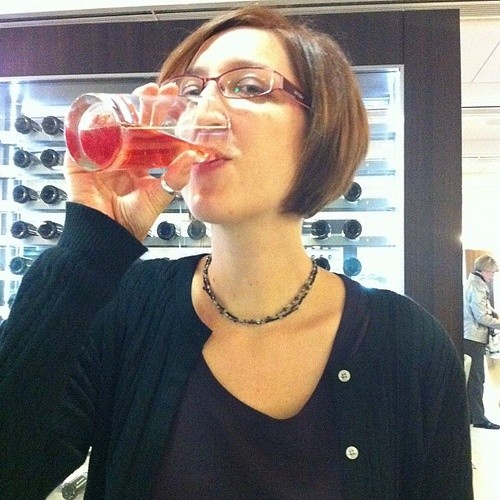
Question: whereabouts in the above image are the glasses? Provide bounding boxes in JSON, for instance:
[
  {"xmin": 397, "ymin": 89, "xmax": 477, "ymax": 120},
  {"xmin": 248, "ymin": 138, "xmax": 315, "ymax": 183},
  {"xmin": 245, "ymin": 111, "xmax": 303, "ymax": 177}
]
[{"xmin": 158, "ymin": 66, "xmax": 311, "ymax": 109}]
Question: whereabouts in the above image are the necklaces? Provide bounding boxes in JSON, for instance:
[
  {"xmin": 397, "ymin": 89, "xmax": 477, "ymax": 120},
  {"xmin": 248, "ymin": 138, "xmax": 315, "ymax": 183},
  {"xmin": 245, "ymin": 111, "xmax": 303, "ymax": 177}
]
[{"xmin": 203, "ymin": 255, "xmax": 318, "ymax": 325}]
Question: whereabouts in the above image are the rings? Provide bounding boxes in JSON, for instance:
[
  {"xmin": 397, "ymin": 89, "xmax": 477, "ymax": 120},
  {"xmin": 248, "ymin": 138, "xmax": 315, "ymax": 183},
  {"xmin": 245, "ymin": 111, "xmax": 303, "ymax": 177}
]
[{"xmin": 161, "ymin": 173, "xmax": 178, "ymax": 195}]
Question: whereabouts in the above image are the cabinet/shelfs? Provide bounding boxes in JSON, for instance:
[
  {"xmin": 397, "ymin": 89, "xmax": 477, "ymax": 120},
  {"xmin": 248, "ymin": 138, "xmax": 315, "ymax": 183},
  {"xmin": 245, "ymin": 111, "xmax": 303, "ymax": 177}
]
[{"xmin": 0, "ymin": 93, "xmax": 398, "ymax": 326}]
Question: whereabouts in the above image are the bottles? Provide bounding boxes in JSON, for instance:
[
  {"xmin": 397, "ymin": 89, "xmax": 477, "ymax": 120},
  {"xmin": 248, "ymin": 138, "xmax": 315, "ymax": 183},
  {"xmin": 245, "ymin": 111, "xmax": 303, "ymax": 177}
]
[
  {"xmin": 343, "ymin": 219, "xmax": 363, "ymax": 241},
  {"xmin": 14, "ymin": 115, "xmax": 41, "ymax": 135},
  {"xmin": 12, "ymin": 185, "xmax": 39, "ymax": 204},
  {"xmin": 61, "ymin": 474, "xmax": 87, "ymax": 500},
  {"xmin": 344, "ymin": 182, "xmax": 362, "ymax": 202},
  {"xmin": 40, "ymin": 149, "xmax": 63, "ymax": 169},
  {"xmin": 343, "ymin": 257, "xmax": 362, "ymax": 276},
  {"xmin": 40, "ymin": 186, "xmax": 67, "ymax": 205},
  {"xmin": 157, "ymin": 221, "xmax": 179, "ymax": 239},
  {"xmin": 188, "ymin": 220, "xmax": 207, "ymax": 239},
  {"xmin": 14, "ymin": 151, "xmax": 40, "ymax": 168},
  {"xmin": 312, "ymin": 258, "xmax": 330, "ymax": 271},
  {"xmin": 9, "ymin": 256, "xmax": 36, "ymax": 275},
  {"xmin": 11, "ymin": 221, "xmax": 38, "ymax": 239},
  {"xmin": 8, "ymin": 293, "xmax": 16, "ymax": 309},
  {"xmin": 311, "ymin": 220, "xmax": 332, "ymax": 241},
  {"xmin": 41, "ymin": 117, "xmax": 63, "ymax": 136},
  {"xmin": 38, "ymin": 221, "xmax": 65, "ymax": 241}
]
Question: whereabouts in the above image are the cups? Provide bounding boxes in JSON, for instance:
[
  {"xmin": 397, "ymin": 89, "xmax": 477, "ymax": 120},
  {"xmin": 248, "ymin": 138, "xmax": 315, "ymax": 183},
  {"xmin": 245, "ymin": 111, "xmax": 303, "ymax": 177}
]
[{"xmin": 63, "ymin": 93, "xmax": 233, "ymax": 174}]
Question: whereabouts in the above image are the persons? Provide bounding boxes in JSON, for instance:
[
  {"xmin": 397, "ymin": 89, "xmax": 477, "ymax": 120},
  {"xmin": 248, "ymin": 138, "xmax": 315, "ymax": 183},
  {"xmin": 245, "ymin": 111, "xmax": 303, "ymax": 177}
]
[
  {"xmin": 0, "ymin": 6, "xmax": 474, "ymax": 500},
  {"xmin": 463, "ymin": 255, "xmax": 500, "ymax": 430}
]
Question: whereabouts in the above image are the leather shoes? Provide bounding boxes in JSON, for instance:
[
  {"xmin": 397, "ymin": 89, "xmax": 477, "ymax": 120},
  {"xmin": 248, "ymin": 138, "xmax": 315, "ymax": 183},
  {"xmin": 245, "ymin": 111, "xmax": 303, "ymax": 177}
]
[{"xmin": 473, "ymin": 419, "xmax": 500, "ymax": 430}]
